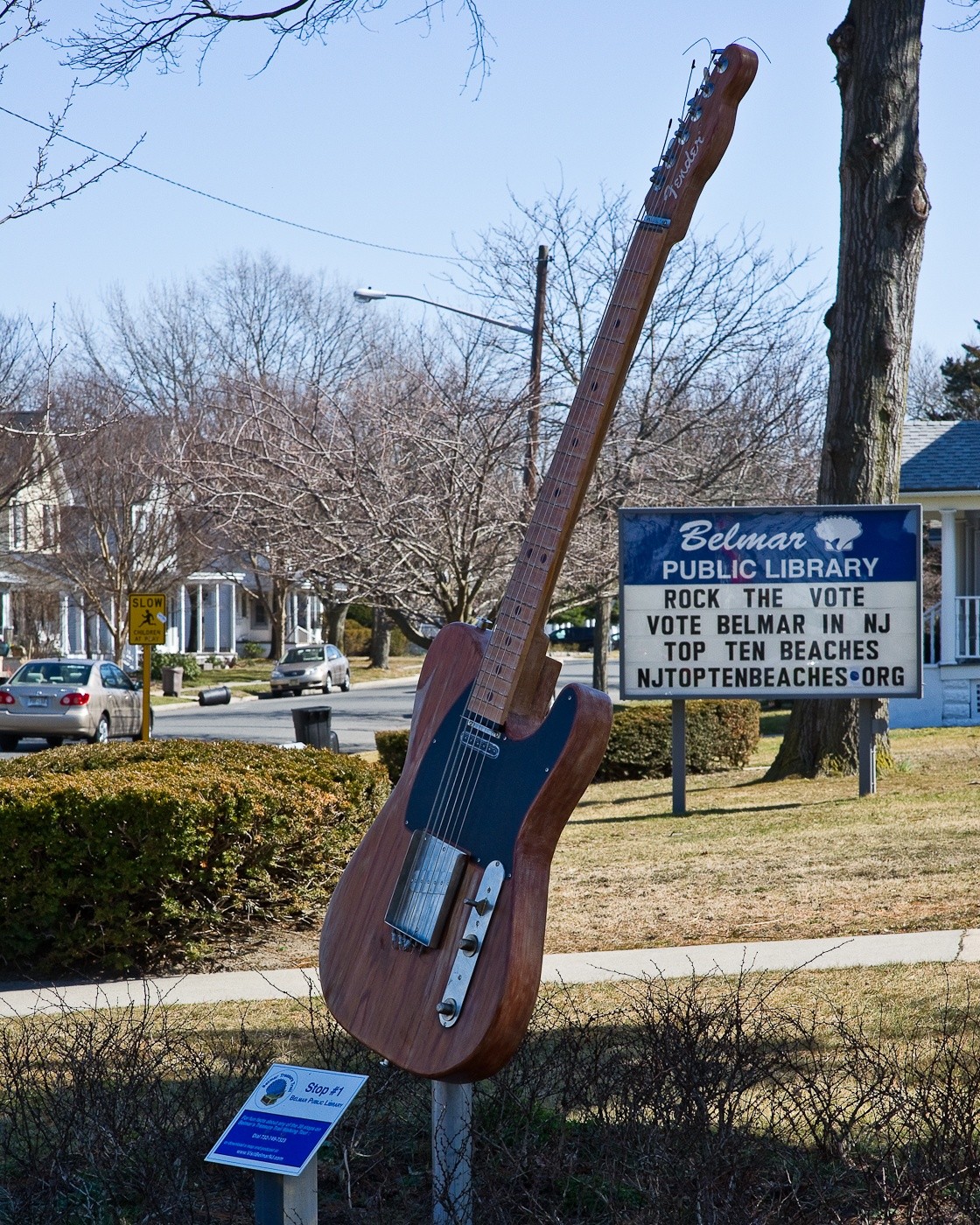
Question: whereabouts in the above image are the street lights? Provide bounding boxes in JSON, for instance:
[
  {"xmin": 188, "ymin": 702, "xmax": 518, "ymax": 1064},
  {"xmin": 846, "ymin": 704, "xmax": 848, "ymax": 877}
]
[{"xmin": 352, "ymin": 289, "xmax": 546, "ymax": 528}]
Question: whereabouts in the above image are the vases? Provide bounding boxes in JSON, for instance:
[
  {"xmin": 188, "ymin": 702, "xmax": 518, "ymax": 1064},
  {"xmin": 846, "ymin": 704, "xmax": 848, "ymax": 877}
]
[
  {"xmin": 0, "ymin": 643, "xmax": 9, "ymax": 656},
  {"xmin": 11, "ymin": 647, "xmax": 23, "ymax": 657}
]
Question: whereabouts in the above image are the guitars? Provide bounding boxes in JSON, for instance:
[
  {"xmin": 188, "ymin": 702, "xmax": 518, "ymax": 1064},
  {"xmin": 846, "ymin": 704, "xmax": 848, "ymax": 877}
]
[{"xmin": 317, "ymin": 42, "xmax": 759, "ymax": 1085}]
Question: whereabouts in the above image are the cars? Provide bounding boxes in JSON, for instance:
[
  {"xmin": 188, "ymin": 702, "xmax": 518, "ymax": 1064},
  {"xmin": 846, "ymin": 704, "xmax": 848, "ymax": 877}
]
[
  {"xmin": 0, "ymin": 659, "xmax": 153, "ymax": 752},
  {"xmin": 549, "ymin": 627, "xmax": 594, "ymax": 652},
  {"xmin": 270, "ymin": 644, "xmax": 350, "ymax": 697}
]
